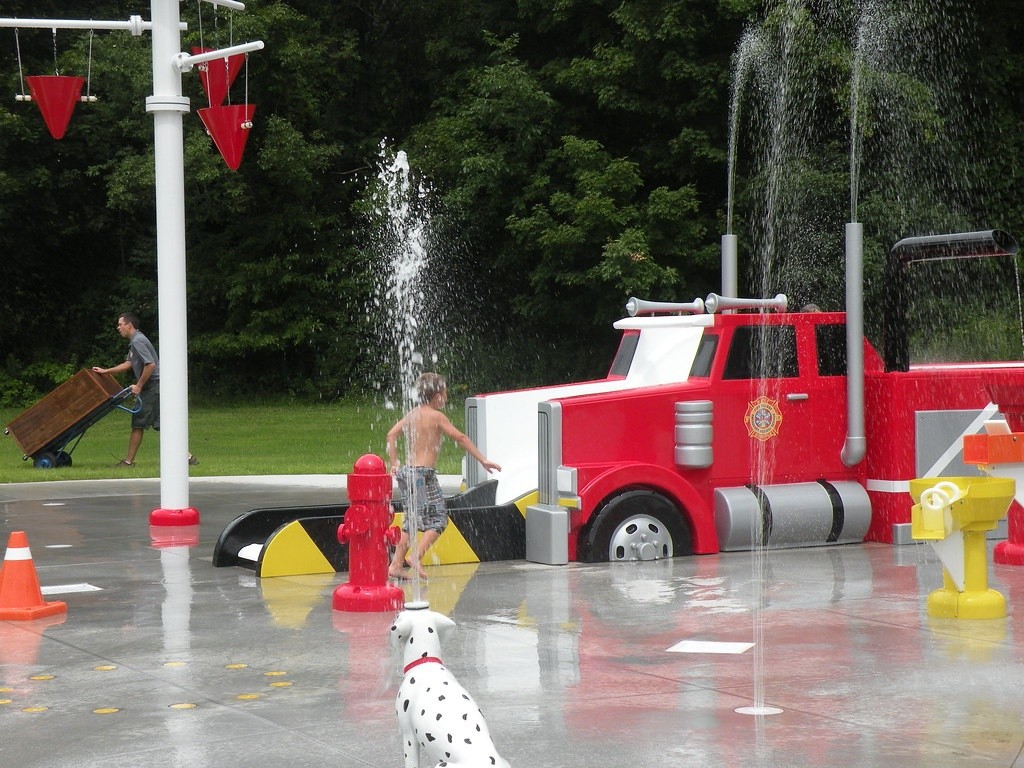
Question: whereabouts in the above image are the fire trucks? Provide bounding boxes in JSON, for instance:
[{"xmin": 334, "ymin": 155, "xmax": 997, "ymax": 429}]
[{"xmin": 459, "ymin": 228, "xmax": 1024, "ymax": 564}]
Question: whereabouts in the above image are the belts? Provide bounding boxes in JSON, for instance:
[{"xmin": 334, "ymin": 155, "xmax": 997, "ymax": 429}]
[{"xmin": 403, "ymin": 463, "xmax": 425, "ymax": 469}]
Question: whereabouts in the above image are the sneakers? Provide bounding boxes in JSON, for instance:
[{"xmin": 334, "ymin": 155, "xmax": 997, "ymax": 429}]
[
  {"xmin": 189, "ymin": 455, "xmax": 201, "ymax": 466},
  {"xmin": 113, "ymin": 458, "xmax": 137, "ymax": 468}
]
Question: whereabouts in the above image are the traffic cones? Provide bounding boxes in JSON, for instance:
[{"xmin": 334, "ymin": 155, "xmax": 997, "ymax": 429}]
[{"xmin": 0, "ymin": 530, "xmax": 68, "ymax": 621}]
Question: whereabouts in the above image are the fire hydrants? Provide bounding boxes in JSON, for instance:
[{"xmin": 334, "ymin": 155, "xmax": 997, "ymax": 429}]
[{"xmin": 331, "ymin": 453, "xmax": 406, "ymax": 612}]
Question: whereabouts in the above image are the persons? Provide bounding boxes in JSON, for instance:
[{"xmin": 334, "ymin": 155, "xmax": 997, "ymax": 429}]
[
  {"xmin": 387, "ymin": 373, "xmax": 502, "ymax": 579},
  {"xmin": 92, "ymin": 312, "xmax": 197, "ymax": 468}
]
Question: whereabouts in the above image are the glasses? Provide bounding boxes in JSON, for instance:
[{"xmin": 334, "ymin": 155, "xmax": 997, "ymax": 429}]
[{"xmin": 116, "ymin": 324, "xmax": 128, "ymax": 330}]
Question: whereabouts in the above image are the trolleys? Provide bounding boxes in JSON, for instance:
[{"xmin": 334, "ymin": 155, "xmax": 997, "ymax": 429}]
[{"xmin": 28, "ymin": 386, "xmax": 143, "ymax": 470}]
